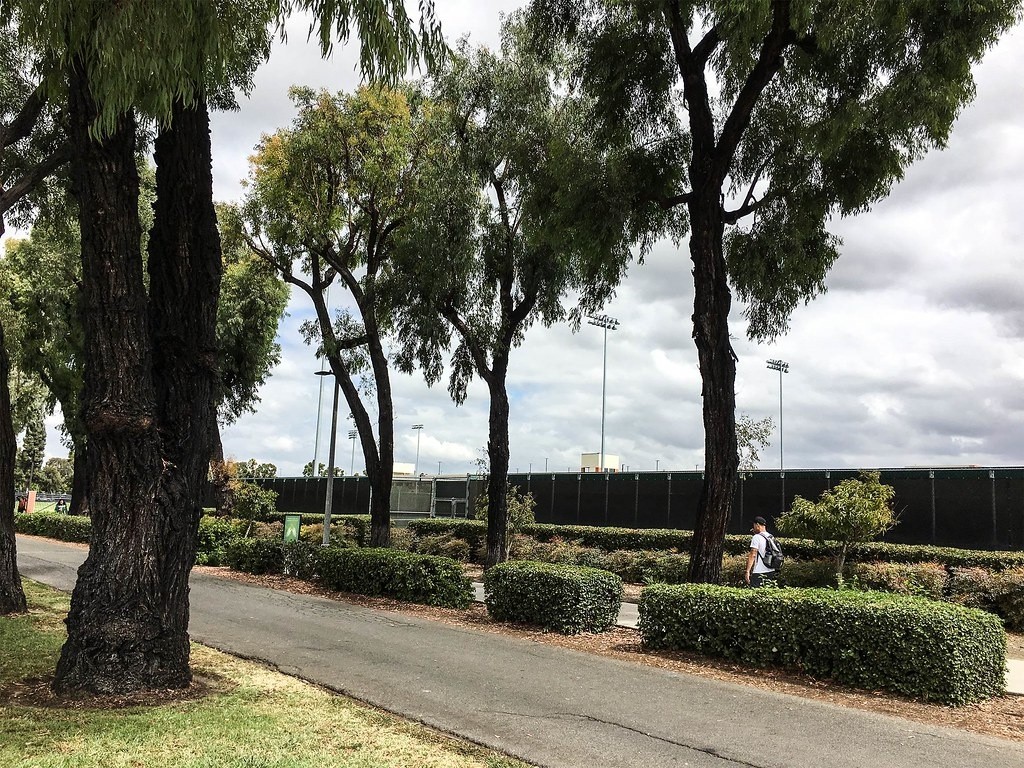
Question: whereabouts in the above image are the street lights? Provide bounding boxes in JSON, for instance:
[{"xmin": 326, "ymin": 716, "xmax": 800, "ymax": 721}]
[
  {"xmin": 314, "ymin": 369, "xmax": 339, "ymax": 549},
  {"xmin": 765, "ymin": 357, "xmax": 790, "ymax": 472},
  {"xmin": 348, "ymin": 429, "xmax": 358, "ymax": 477},
  {"xmin": 586, "ymin": 312, "xmax": 621, "ymax": 472},
  {"xmin": 412, "ymin": 423, "xmax": 424, "ymax": 478}
]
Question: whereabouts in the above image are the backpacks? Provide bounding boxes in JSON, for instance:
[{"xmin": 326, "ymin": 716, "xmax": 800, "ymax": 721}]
[{"xmin": 757, "ymin": 533, "xmax": 785, "ymax": 569}]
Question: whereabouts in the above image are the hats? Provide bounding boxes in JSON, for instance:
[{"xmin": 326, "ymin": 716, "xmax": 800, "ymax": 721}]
[{"xmin": 749, "ymin": 517, "xmax": 766, "ymax": 525}]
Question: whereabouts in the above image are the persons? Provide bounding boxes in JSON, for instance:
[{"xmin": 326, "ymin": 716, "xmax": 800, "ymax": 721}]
[
  {"xmin": 54, "ymin": 499, "xmax": 67, "ymax": 514},
  {"xmin": 745, "ymin": 516, "xmax": 776, "ymax": 588},
  {"xmin": 18, "ymin": 496, "xmax": 25, "ymax": 513}
]
